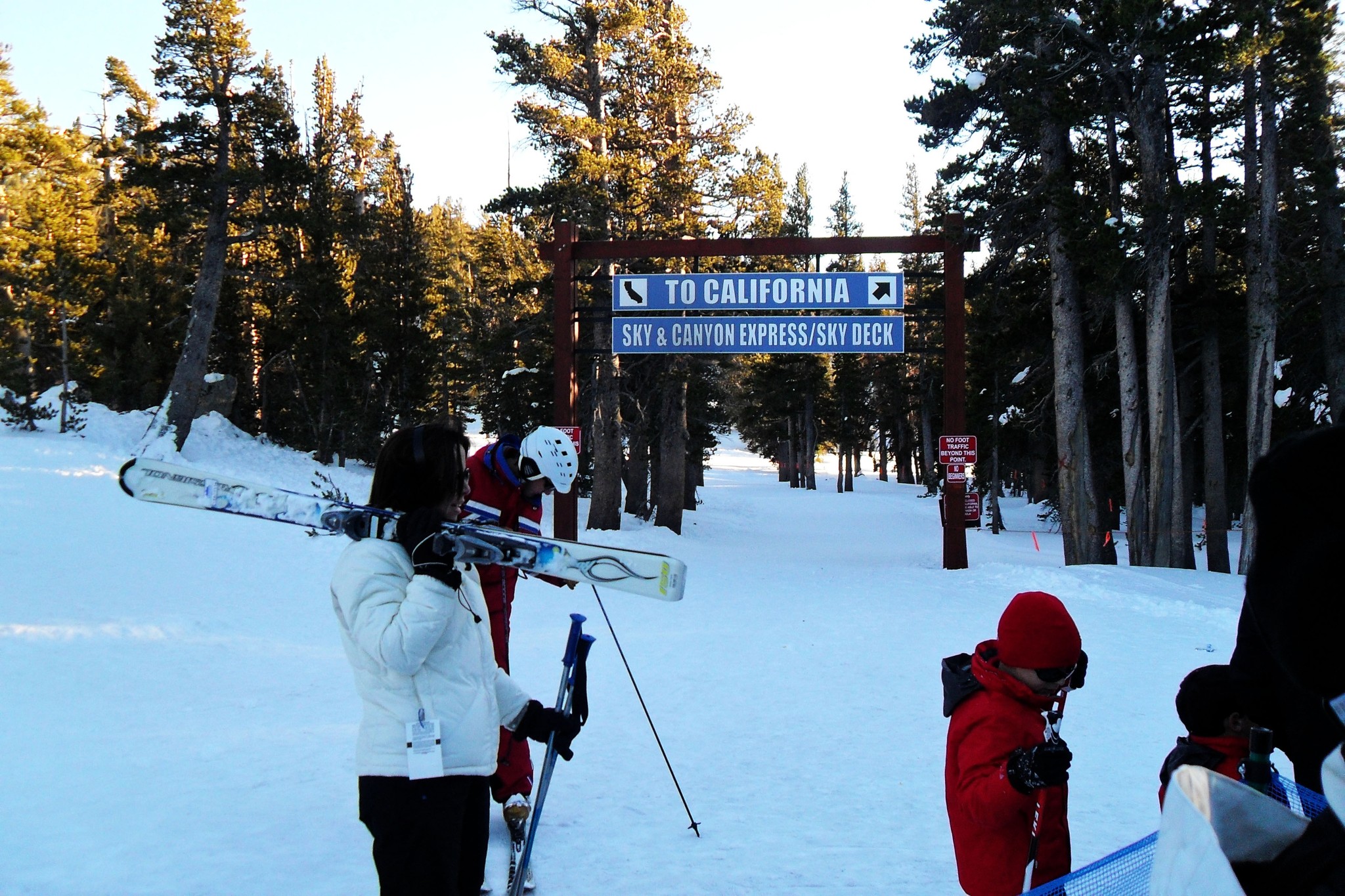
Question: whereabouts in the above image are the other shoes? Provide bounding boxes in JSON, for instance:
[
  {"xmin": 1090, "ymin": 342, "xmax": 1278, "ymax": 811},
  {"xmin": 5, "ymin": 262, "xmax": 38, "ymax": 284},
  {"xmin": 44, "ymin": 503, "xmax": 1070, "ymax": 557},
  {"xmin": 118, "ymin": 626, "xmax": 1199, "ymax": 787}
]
[{"xmin": 504, "ymin": 793, "xmax": 533, "ymax": 822}]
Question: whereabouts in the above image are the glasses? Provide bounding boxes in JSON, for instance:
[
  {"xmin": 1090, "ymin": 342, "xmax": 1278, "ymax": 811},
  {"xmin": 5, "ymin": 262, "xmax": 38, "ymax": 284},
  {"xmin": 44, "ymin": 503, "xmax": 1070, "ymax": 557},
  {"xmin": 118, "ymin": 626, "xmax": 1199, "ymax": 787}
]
[
  {"xmin": 1035, "ymin": 667, "xmax": 1078, "ymax": 683},
  {"xmin": 458, "ymin": 467, "xmax": 472, "ymax": 482}
]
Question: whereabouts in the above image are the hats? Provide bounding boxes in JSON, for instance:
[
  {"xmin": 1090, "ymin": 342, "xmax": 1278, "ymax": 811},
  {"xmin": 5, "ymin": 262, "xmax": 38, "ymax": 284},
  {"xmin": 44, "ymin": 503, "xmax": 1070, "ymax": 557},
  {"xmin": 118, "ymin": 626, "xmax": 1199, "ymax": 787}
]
[{"xmin": 997, "ymin": 591, "xmax": 1083, "ymax": 667}]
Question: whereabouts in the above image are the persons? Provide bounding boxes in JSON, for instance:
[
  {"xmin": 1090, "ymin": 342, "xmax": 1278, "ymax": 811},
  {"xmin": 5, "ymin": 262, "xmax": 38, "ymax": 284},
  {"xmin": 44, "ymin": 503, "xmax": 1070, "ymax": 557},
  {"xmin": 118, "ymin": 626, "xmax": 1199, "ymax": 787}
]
[
  {"xmin": 938, "ymin": 592, "xmax": 1088, "ymax": 896},
  {"xmin": 1159, "ymin": 665, "xmax": 1290, "ymax": 815},
  {"xmin": 465, "ymin": 425, "xmax": 579, "ymax": 825},
  {"xmin": 335, "ymin": 423, "xmax": 581, "ymax": 896}
]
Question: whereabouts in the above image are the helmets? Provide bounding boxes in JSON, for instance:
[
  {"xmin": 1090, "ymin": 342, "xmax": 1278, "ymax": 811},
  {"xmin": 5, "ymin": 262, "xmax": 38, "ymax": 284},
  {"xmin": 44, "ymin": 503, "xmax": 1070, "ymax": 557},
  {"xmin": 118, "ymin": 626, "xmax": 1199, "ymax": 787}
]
[{"xmin": 519, "ymin": 426, "xmax": 579, "ymax": 494}]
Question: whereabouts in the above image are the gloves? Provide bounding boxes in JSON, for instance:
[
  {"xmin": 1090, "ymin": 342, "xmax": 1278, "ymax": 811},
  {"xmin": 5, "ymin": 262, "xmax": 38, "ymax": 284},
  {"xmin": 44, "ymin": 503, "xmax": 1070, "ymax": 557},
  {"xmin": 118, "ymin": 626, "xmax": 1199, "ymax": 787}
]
[
  {"xmin": 1066, "ymin": 650, "xmax": 1089, "ymax": 690},
  {"xmin": 513, "ymin": 699, "xmax": 580, "ymax": 761},
  {"xmin": 1007, "ymin": 737, "xmax": 1073, "ymax": 795},
  {"xmin": 396, "ymin": 504, "xmax": 463, "ymax": 592}
]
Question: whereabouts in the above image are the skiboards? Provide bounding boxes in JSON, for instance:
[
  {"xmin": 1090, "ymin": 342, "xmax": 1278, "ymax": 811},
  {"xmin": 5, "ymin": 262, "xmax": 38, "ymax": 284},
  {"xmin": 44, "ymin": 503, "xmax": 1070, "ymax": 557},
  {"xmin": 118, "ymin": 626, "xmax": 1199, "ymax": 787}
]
[
  {"xmin": 481, "ymin": 806, "xmax": 538, "ymax": 894},
  {"xmin": 118, "ymin": 455, "xmax": 686, "ymax": 602}
]
